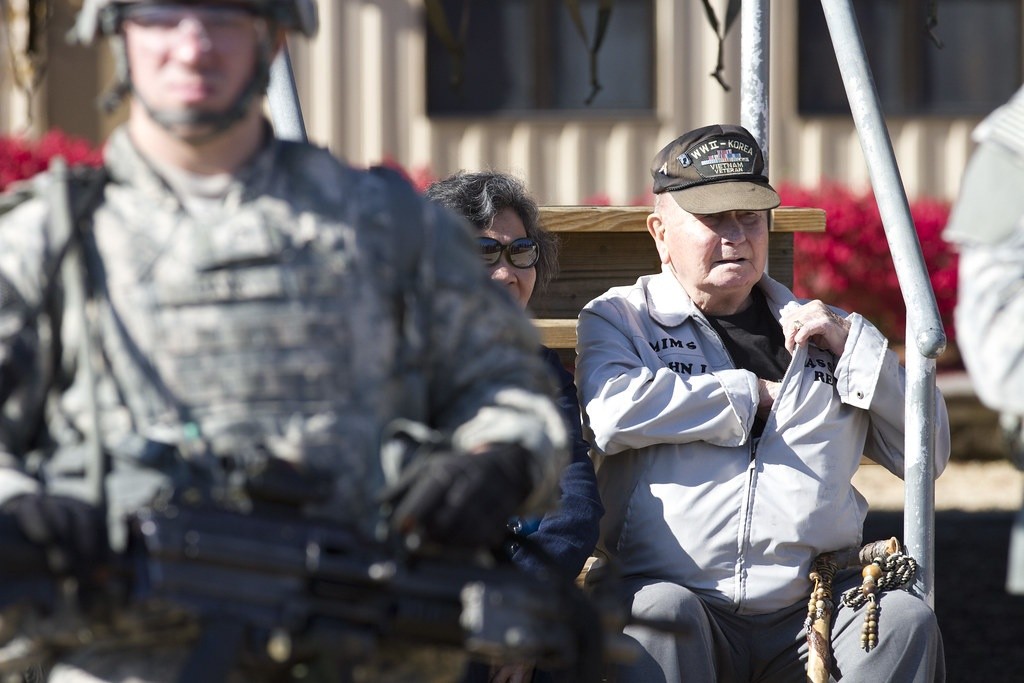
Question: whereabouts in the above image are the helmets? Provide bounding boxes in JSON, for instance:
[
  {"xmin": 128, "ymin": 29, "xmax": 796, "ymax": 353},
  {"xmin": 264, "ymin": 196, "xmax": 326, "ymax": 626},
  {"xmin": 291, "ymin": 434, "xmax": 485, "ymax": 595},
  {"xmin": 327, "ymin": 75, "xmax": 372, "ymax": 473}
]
[{"xmin": 76, "ymin": 0, "xmax": 320, "ymax": 46}]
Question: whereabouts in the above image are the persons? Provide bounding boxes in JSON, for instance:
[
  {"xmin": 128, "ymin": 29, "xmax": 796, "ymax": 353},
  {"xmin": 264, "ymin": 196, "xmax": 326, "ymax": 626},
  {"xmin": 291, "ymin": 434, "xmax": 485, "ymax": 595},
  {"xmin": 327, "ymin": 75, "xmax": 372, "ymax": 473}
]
[
  {"xmin": 419, "ymin": 166, "xmax": 610, "ymax": 683},
  {"xmin": 0, "ymin": 1, "xmax": 574, "ymax": 683},
  {"xmin": 940, "ymin": 78, "xmax": 1024, "ymax": 599},
  {"xmin": 570, "ymin": 122, "xmax": 954, "ymax": 683}
]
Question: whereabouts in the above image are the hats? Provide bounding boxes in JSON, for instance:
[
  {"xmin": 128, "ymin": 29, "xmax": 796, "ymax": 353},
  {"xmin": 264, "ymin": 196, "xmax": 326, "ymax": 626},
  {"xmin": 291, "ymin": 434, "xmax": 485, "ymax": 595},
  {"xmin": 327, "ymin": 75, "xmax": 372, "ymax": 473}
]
[{"xmin": 650, "ymin": 125, "xmax": 780, "ymax": 214}]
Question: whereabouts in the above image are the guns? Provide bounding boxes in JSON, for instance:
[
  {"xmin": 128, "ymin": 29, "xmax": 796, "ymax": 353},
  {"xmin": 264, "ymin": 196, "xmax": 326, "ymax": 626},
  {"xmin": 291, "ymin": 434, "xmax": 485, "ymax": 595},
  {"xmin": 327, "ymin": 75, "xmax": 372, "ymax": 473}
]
[{"xmin": 36, "ymin": 433, "xmax": 695, "ymax": 681}]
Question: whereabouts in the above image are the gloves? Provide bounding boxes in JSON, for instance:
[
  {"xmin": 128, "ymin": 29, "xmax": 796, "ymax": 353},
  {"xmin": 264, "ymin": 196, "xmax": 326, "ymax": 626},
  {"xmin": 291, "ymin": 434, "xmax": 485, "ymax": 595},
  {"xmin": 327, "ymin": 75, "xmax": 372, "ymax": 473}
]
[
  {"xmin": 382, "ymin": 442, "xmax": 536, "ymax": 559},
  {"xmin": 4, "ymin": 496, "xmax": 113, "ymax": 606}
]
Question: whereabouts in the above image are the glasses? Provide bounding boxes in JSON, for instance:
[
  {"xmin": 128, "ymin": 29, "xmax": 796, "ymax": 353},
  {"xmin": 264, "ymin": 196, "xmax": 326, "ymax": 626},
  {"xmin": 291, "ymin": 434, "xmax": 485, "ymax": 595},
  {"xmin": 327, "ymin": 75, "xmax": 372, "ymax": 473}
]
[{"xmin": 478, "ymin": 235, "xmax": 540, "ymax": 269}]
